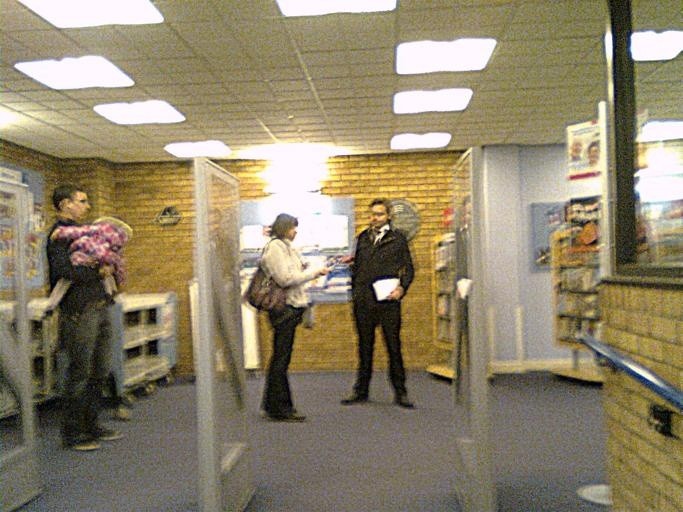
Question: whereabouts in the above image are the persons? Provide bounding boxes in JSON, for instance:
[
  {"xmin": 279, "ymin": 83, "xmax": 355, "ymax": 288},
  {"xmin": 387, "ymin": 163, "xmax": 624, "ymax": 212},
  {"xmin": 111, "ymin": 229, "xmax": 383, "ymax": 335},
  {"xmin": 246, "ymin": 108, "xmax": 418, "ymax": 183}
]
[
  {"xmin": 35, "ymin": 214, "xmax": 133, "ymax": 315},
  {"xmin": 255, "ymin": 213, "xmax": 334, "ymax": 421},
  {"xmin": 586, "ymin": 140, "xmax": 600, "ymax": 165},
  {"xmin": 569, "ymin": 137, "xmax": 585, "ymax": 163},
  {"xmin": 337, "ymin": 196, "xmax": 417, "ymax": 408},
  {"xmin": 43, "ymin": 184, "xmax": 125, "ymax": 451},
  {"xmin": 448, "ymin": 194, "xmax": 474, "ymax": 406}
]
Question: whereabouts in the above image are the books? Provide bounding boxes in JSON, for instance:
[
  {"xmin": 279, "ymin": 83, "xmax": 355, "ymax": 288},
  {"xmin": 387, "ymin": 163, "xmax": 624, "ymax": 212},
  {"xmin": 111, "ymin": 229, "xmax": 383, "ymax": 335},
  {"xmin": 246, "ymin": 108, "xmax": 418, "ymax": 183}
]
[{"xmin": 372, "ymin": 277, "xmax": 405, "ymax": 301}]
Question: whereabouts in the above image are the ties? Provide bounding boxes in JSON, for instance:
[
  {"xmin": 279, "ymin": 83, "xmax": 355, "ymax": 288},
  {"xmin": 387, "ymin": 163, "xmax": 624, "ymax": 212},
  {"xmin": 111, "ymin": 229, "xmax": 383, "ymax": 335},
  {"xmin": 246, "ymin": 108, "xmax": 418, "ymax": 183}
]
[{"xmin": 371, "ymin": 231, "xmax": 378, "ymax": 244}]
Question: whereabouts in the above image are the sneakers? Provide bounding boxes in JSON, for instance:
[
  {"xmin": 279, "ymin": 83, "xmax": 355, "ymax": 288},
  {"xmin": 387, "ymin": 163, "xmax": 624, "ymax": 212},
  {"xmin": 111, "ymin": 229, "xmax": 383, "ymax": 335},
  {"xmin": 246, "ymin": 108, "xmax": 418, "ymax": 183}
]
[
  {"xmin": 395, "ymin": 395, "xmax": 413, "ymax": 408},
  {"xmin": 340, "ymin": 388, "xmax": 369, "ymax": 403},
  {"xmin": 259, "ymin": 407, "xmax": 306, "ymax": 422},
  {"xmin": 73, "ymin": 441, "xmax": 100, "ymax": 451},
  {"xmin": 99, "ymin": 431, "xmax": 124, "ymax": 440}
]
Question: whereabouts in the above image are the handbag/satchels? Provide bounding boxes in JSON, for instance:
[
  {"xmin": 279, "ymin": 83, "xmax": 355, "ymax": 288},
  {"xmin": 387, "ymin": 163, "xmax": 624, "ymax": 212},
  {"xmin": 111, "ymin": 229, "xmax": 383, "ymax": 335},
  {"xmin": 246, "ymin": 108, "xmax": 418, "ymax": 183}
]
[{"xmin": 246, "ymin": 269, "xmax": 286, "ymax": 312}]
